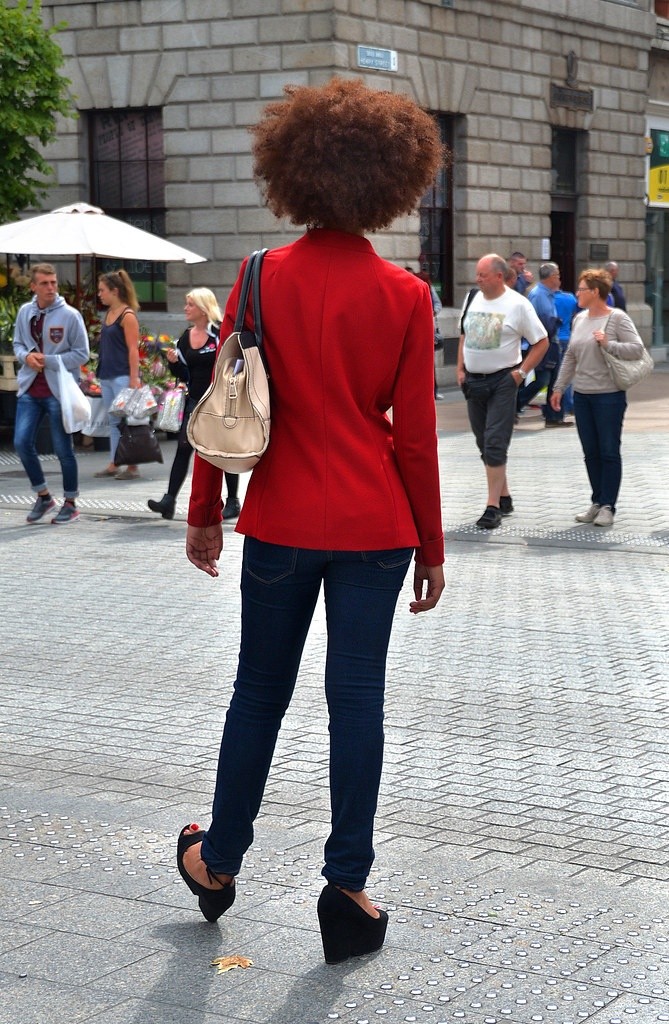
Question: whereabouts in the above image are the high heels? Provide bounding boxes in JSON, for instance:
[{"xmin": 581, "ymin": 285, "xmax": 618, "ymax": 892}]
[
  {"xmin": 176, "ymin": 823, "xmax": 236, "ymax": 924},
  {"xmin": 317, "ymin": 884, "xmax": 389, "ymax": 965}
]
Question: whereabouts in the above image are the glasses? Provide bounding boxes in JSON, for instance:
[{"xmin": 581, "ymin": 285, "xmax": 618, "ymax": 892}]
[{"xmin": 577, "ymin": 287, "xmax": 593, "ymax": 292}]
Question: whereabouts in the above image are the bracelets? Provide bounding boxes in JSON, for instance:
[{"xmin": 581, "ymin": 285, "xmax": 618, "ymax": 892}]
[{"xmin": 517, "ymin": 370, "xmax": 527, "ymax": 379}]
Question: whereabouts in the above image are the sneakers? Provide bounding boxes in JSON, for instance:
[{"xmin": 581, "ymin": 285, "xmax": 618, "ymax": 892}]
[
  {"xmin": 51, "ymin": 502, "xmax": 80, "ymax": 523},
  {"xmin": 575, "ymin": 502, "xmax": 601, "ymax": 523},
  {"xmin": 594, "ymin": 504, "xmax": 615, "ymax": 526},
  {"xmin": 27, "ymin": 496, "xmax": 56, "ymax": 521},
  {"xmin": 500, "ymin": 496, "xmax": 512, "ymax": 512},
  {"xmin": 476, "ymin": 505, "xmax": 502, "ymax": 528}
]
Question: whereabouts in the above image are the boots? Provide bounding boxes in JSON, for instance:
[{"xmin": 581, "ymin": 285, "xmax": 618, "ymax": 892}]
[
  {"xmin": 222, "ymin": 498, "xmax": 241, "ymax": 518},
  {"xmin": 149, "ymin": 494, "xmax": 176, "ymax": 519}
]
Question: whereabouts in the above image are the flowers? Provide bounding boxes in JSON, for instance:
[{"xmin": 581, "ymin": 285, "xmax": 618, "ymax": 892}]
[{"xmin": 0, "ymin": 263, "xmax": 188, "ymax": 398}]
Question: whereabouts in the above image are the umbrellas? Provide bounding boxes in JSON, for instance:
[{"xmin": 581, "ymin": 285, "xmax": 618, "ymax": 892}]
[{"xmin": 0, "ymin": 201, "xmax": 211, "ymax": 312}]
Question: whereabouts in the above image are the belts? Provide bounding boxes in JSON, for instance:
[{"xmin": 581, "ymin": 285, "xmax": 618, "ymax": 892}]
[{"xmin": 464, "ymin": 367, "xmax": 510, "ymax": 379}]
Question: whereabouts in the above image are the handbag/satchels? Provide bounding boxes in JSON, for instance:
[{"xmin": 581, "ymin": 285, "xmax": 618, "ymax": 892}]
[
  {"xmin": 56, "ymin": 353, "xmax": 92, "ymax": 434},
  {"xmin": 155, "ymin": 366, "xmax": 190, "ymax": 430},
  {"xmin": 187, "ymin": 246, "xmax": 271, "ymax": 475},
  {"xmin": 134, "ymin": 385, "xmax": 159, "ymax": 418},
  {"xmin": 114, "ymin": 416, "xmax": 164, "ymax": 466},
  {"xmin": 107, "ymin": 386, "xmax": 143, "ymax": 416},
  {"xmin": 600, "ymin": 308, "xmax": 654, "ymax": 391}
]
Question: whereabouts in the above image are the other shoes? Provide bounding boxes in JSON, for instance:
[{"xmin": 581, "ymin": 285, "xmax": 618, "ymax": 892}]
[
  {"xmin": 545, "ymin": 419, "xmax": 574, "ymax": 428},
  {"xmin": 94, "ymin": 468, "xmax": 122, "ymax": 478},
  {"xmin": 116, "ymin": 471, "xmax": 141, "ymax": 479}
]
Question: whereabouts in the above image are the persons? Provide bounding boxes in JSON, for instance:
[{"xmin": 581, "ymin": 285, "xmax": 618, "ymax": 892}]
[
  {"xmin": 178, "ymin": 76, "xmax": 446, "ymax": 965},
  {"xmin": 404, "ymin": 266, "xmax": 445, "ymax": 400},
  {"xmin": 148, "ymin": 287, "xmax": 242, "ymax": 521},
  {"xmin": 12, "ymin": 262, "xmax": 91, "ymax": 524},
  {"xmin": 457, "ymin": 253, "xmax": 550, "ymax": 529},
  {"xmin": 93, "ymin": 267, "xmax": 142, "ymax": 480},
  {"xmin": 549, "ymin": 269, "xmax": 645, "ymax": 526},
  {"xmin": 502, "ymin": 250, "xmax": 628, "ymax": 428}
]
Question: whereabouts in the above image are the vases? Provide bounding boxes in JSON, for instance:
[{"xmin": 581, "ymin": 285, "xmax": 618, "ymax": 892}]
[
  {"xmin": 165, "ymin": 430, "xmax": 179, "ymax": 441},
  {"xmin": 93, "ymin": 436, "xmax": 110, "ymax": 453}
]
